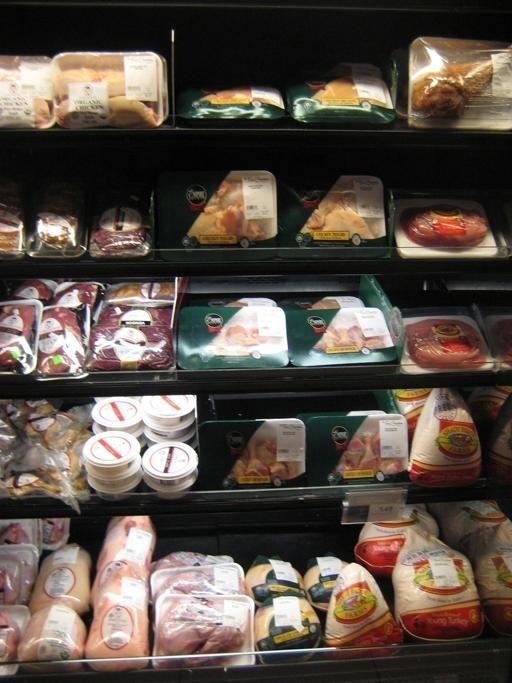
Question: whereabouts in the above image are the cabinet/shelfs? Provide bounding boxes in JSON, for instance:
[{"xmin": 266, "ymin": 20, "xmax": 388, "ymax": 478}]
[{"xmin": 1, "ymin": 127, "xmax": 512, "ymax": 682}]
